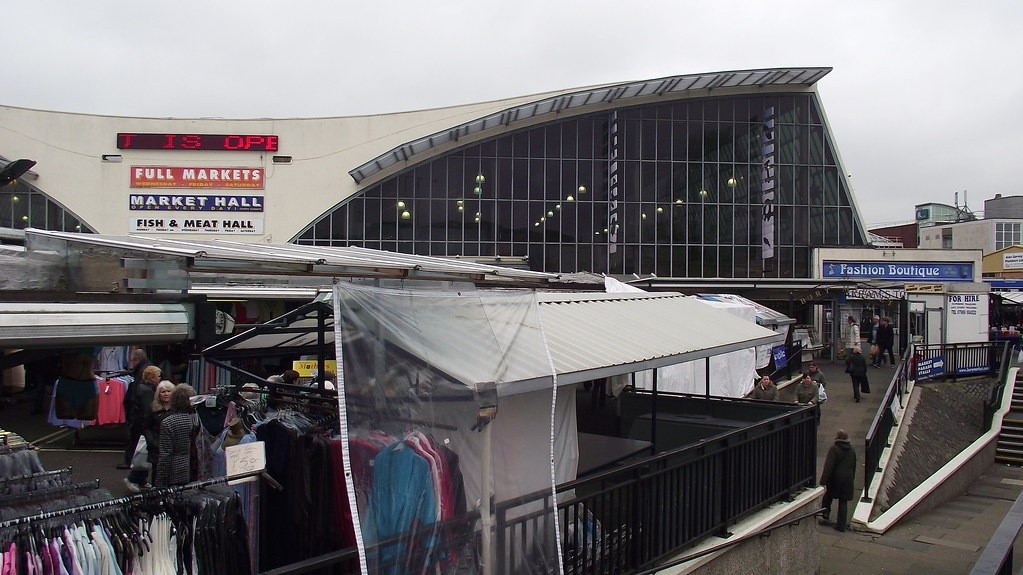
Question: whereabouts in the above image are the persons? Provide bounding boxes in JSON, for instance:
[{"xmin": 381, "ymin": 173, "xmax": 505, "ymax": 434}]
[
  {"xmin": 236, "ymin": 369, "xmax": 336, "ymax": 407},
  {"xmin": 872, "ymin": 317, "xmax": 896, "ymax": 368},
  {"xmin": 142, "ymin": 381, "xmax": 175, "ymax": 485},
  {"xmin": 845, "ymin": 346, "xmax": 867, "ymax": 402},
  {"xmin": 117, "ymin": 349, "xmax": 149, "ymax": 469},
  {"xmin": 154, "ymin": 383, "xmax": 203, "ymax": 489},
  {"xmin": 867, "ymin": 315, "xmax": 887, "ymax": 366},
  {"xmin": 125, "ymin": 366, "xmax": 161, "ymax": 493},
  {"xmin": 819, "ymin": 430, "xmax": 856, "ymax": 532},
  {"xmin": 793, "ymin": 373, "xmax": 819, "ymax": 404},
  {"xmin": 805, "ymin": 362, "xmax": 827, "ymax": 425},
  {"xmin": 845, "ymin": 315, "xmax": 861, "ymax": 373},
  {"xmin": 751, "ymin": 374, "xmax": 779, "ymax": 401}
]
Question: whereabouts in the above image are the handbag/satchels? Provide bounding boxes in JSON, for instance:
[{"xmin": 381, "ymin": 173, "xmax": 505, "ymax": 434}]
[
  {"xmin": 845, "ymin": 361, "xmax": 855, "ymax": 374},
  {"xmin": 869, "ymin": 344, "xmax": 879, "ymax": 360},
  {"xmin": 817, "ymin": 383, "xmax": 827, "ymax": 404}
]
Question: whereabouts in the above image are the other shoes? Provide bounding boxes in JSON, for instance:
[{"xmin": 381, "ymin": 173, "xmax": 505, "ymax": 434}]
[
  {"xmin": 856, "ymin": 397, "xmax": 860, "ymax": 403},
  {"xmin": 873, "ymin": 363, "xmax": 880, "ymax": 369},
  {"xmin": 837, "ymin": 525, "xmax": 845, "ymax": 532},
  {"xmin": 124, "ymin": 478, "xmax": 140, "ymax": 492},
  {"xmin": 891, "ymin": 364, "xmax": 896, "ymax": 368},
  {"xmin": 142, "ymin": 483, "xmax": 152, "ymax": 489},
  {"xmin": 116, "ymin": 464, "xmax": 131, "ymax": 469}
]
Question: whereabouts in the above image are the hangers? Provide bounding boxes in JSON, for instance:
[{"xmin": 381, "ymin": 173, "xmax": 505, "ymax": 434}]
[
  {"xmin": 0, "ymin": 476, "xmax": 242, "ymax": 560},
  {"xmin": 220, "ymin": 397, "xmax": 432, "ymax": 453}
]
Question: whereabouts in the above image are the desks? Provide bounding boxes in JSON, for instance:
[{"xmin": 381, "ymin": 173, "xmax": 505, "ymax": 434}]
[{"xmin": 577, "ymin": 432, "xmax": 654, "ymax": 479}]
